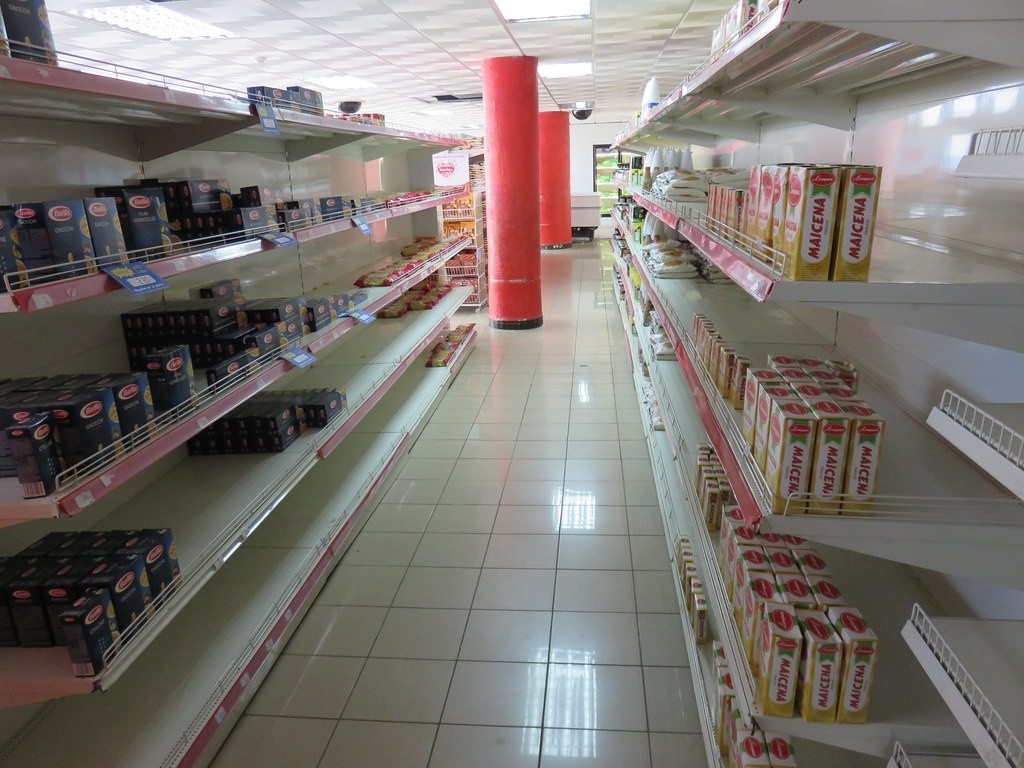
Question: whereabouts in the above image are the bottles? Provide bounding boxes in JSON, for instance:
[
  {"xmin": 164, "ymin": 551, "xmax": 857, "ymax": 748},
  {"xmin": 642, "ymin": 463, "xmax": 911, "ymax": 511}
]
[
  {"xmin": 641, "ymin": 77, "xmax": 661, "ymax": 117},
  {"xmin": 640, "ymin": 146, "xmax": 695, "ymax": 196}
]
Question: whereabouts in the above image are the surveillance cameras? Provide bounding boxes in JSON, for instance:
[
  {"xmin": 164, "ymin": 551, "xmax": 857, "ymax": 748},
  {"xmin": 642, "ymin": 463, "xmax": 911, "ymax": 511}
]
[
  {"xmin": 338, "ymin": 101, "xmax": 361, "ymax": 113},
  {"xmin": 572, "ymin": 110, "xmax": 592, "ymax": 120}
]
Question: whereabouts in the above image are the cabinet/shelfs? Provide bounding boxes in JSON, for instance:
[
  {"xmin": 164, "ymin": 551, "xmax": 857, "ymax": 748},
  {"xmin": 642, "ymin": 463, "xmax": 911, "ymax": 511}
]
[
  {"xmin": 601, "ymin": 0, "xmax": 1023, "ymax": 768},
  {"xmin": 0, "ymin": 35, "xmax": 478, "ymax": 768},
  {"xmin": 439, "ymin": 188, "xmax": 485, "ymax": 311},
  {"xmin": 432, "ymin": 146, "xmax": 488, "ymax": 313},
  {"xmin": 886, "ymin": 130, "xmax": 1023, "ymax": 768}
]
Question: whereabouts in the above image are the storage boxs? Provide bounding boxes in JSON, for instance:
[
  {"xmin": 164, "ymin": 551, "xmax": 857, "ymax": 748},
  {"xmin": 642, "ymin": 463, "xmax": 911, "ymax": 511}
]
[
  {"xmin": 246, "ymin": 85, "xmax": 386, "ymax": 126},
  {"xmin": 0, "ymin": 527, "xmax": 184, "ymax": 679},
  {"xmin": 676, "ymin": 163, "xmax": 885, "ymax": 768},
  {"xmin": 710, "ymin": 0, "xmax": 780, "ymax": 61},
  {"xmin": 0, "ymin": 176, "xmax": 376, "ymax": 498},
  {"xmin": 0, "ymin": 0, "xmax": 61, "ymax": 69}
]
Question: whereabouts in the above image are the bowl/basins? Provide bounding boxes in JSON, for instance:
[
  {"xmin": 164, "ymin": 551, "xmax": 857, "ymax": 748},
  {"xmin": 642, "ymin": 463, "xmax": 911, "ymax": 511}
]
[{"xmin": 338, "ymin": 101, "xmax": 362, "ymax": 114}]
